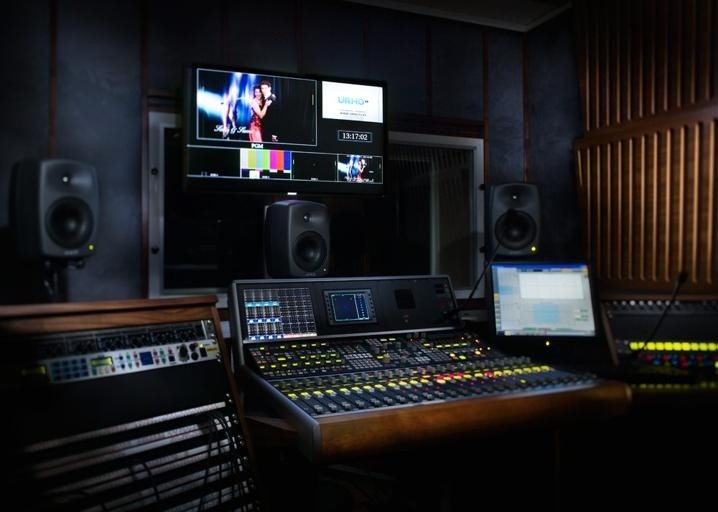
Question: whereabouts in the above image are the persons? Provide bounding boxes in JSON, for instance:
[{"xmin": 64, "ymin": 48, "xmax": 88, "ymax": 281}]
[
  {"xmin": 221, "ymin": 97, "xmax": 240, "ymax": 136},
  {"xmin": 248, "ymin": 81, "xmax": 280, "ymax": 149},
  {"xmin": 357, "ymin": 158, "xmax": 368, "ymax": 180}
]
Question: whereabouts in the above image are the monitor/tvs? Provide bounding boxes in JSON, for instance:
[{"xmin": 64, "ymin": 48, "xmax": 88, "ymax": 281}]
[
  {"xmin": 486, "ymin": 260, "xmax": 598, "ymax": 338},
  {"xmin": 182, "ymin": 62, "xmax": 389, "ymax": 198}
]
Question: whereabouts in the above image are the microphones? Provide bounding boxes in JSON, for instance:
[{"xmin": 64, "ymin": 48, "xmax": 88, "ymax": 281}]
[{"xmin": 634, "ymin": 272, "xmax": 688, "ymax": 365}]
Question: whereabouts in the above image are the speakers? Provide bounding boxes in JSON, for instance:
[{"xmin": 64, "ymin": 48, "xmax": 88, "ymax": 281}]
[
  {"xmin": 488, "ymin": 182, "xmax": 541, "ymax": 260},
  {"xmin": 264, "ymin": 201, "xmax": 332, "ymax": 279},
  {"xmin": 9, "ymin": 159, "xmax": 100, "ymax": 260}
]
